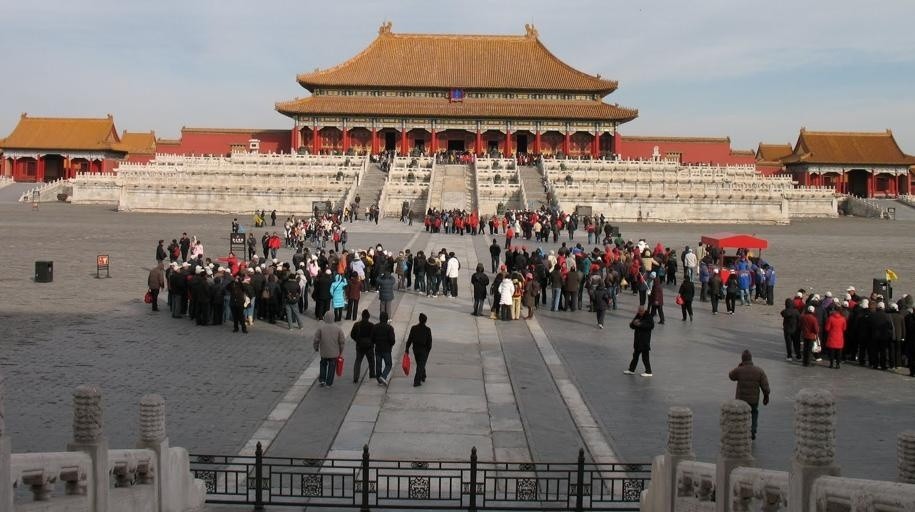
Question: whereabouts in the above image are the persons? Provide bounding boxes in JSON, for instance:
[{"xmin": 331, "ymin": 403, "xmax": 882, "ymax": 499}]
[
  {"xmin": 729, "ymin": 348, "xmax": 771, "ymax": 442},
  {"xmin": 626, "ymin": 306, "xmax": 655, "ymax": 377},
  {"xmin": 614, "ymin": 232, "xmax": 775, "ymax": 324},
  {"xmin": 405, "ymin": 311, "xmax": 434, "ymax": 388},
  {"xmin": 350, "ymin": 308, "xmax": 376, "ymax": 384},
  {"xmin": 780, "ymin": 286, "xmax": 913, "ymax": 377},
  {"xmin": 228, "ymin": 194, "xmax": 624, "ymax": 335},
  {"xmin": 147, "ymin": 232, "xmax": 238, "ymax": 326},
  {"xmin": 541, "ymin": 176, "xmax": 552, "ymax": 205},
  {"xmin": 312, "ymin": 310, "xmax": 346, "ymax": 387},
  {"xmin": 682, "ymin": 160, "xmax": 713, "ymax": 166},
  {"xmin": 298, "ymin": 144, "xmax": 649, "ymax": 171},
  {"xmin": 203, "ymin": 152, "xmax": 216, "ymax": 157},
  {"xmin": 372, "ymin": 312, "xmax": 397, "ymax": 387}
]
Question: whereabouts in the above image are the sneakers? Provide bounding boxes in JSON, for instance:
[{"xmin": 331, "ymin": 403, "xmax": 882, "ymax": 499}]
[
  {"xmin": 402, "ymin": 352, "xmax": 411, "ymax": 376},
  {"xmin": 398, "ymin": 286, "xmax": 456, "ymax": 298},
  {"xmin": 598, "ymin": 324, "xmax": 603, "ymax": 329},
  {"xmin": 624, "ymin": 370, "xmax": 635, "ymax": 375},
  {"xmin": 379, "ymin": 376, "xmax": 388, "ymax": 387},
  {"xmin": 641, "ymin": 372, "xmax": 652, "ymax": 376}
]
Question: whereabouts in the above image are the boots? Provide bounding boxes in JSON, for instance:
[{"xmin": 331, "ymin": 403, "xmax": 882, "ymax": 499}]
[{"xmin": 490, "ymin": 311, "xmax": 497, "ymax": 319}]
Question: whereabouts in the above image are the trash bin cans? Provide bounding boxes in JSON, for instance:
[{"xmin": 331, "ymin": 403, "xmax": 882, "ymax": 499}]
[
  {"xmin": 24, "ymin": 196, "xmax": 29, "ymax": 201},
  {"xmin": 35, "ymin": 261, "xmax": 53, "ymax": 283},
  {"xmin": 873, "ymin": 279, "xmax": 892, "ymax": 299},
  {"xmin": 883, "ymin": 212, "xmax": 888, "ymax": 220}
]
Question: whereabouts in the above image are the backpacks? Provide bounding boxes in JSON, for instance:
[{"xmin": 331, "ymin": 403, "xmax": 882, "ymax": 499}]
[
  {"xmin": 513, "ymin": 281, "xmax": 521, "ymax": 297},
  {"xmin": 286, "ymin": 290, "xmax": 300, "ymax": 304},
  {"xmin": 531, "ymin": 280, "xmax": 540, "ymax": 297}
]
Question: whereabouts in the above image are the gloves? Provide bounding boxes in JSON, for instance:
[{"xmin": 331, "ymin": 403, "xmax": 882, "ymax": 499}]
[{"xmin": 763, "ymin": 394, "xmax": 769, "ymax": 405}]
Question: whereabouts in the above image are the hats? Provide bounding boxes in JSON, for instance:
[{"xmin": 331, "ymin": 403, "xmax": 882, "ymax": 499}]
[
  {"xmin": 169, "ymin": 254, "xmax": 331, "ymax": 275},
  {"xmin": 354, "ymin": 246, "xmax": 392, "ymax": 260},
  {"xmin": 796, "ymin": 285, "xmax": 913, "ymax": 314},
  {"xmin": 501, "ymin": 246, "xmax": 533, "ymax": 279}
]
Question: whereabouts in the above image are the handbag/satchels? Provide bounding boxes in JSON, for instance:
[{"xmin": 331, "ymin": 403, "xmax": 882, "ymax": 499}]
[
  {"xmin": 261, "ymin": 287, "xmax": 270, "ymax": 299},
  {"xmin": 336, "ymin": 353, "xmax": 344, "ymax": 376},
  {"xmin": 812, "ymin": 337, "xmax": 821, "ymax": 353},
  {"xmin": 676, "ymin": 294, "xmax": 684, "ymax": 305},
  {"xmin": 144, "ymin": 288, "xmax": 153, "ymax": 303}
]
